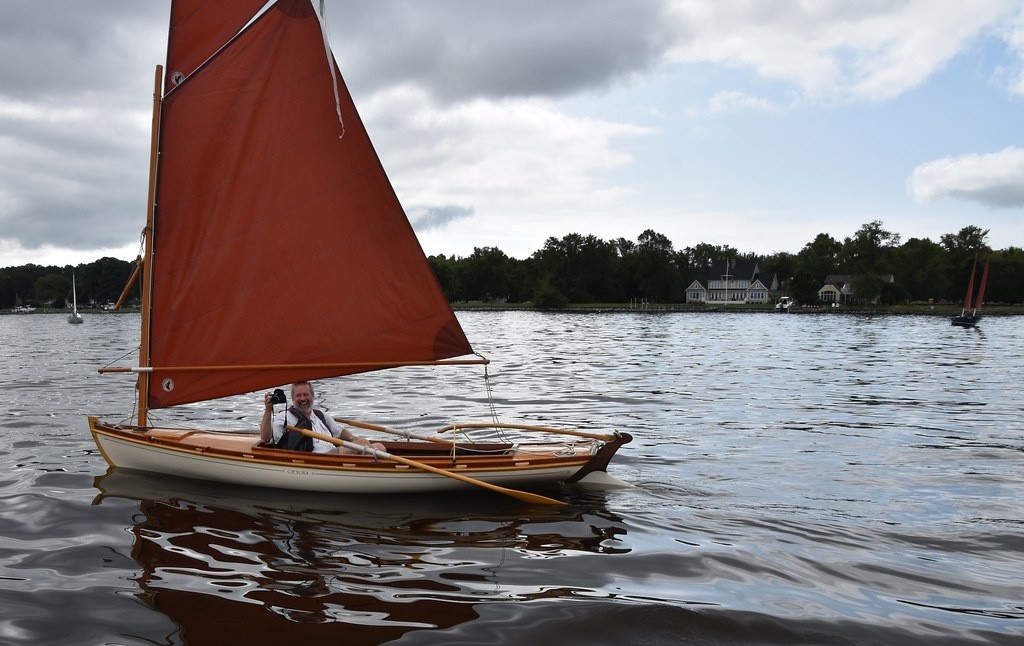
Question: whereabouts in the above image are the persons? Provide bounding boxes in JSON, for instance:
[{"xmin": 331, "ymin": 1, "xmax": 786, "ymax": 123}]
[
  {"xmin": 779, "ymin": 299, "xmax": 786, "ymax": 312},
  {"xmin": 259, "ymin": 380, "xmax": 385, "ymax": 454}
]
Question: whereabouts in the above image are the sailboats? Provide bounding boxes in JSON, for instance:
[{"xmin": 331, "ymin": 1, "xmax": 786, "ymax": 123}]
[
  {"xmin": 949, "ymin": 240, "xmax": 992, "ymax": 328},
  {"xmin": 83, "ymin": 1, "xmax": 635, "ymax": 496},
  {"xmin": 67, "ymin": 272, "xmax": 84, "ymax": 324}
]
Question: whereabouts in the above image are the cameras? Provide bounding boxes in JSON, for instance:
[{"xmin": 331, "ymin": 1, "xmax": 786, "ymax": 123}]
[{"xmin": 270, "ymin": 389, "xmax": 287, "ymax": 404}]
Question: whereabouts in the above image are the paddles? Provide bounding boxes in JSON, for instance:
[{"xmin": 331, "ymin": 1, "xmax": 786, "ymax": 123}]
[
  {"xmin": 285, "ymin": 422, "xmax": 564, "ymax": 505},
  {"xmin": 333, "ymin": 416, "xmax": 449, "ymax": 444}
]
